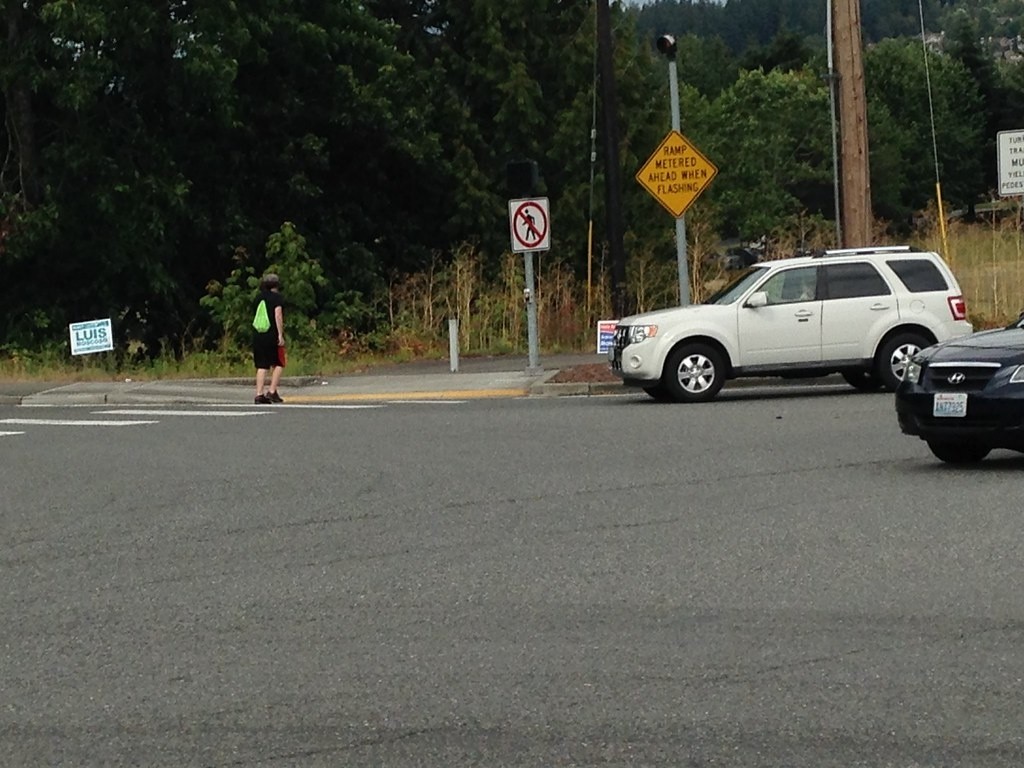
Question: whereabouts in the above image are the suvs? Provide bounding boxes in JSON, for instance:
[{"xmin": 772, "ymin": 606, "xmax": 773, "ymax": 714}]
[{"xmin": 610, "ymin": 244, "xmax": 973, "ymax": 403}]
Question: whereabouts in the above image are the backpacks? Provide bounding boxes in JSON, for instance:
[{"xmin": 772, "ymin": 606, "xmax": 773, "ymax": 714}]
[{"xmin": 252, "ymin": 300, "xmax": 271, "ymax": 333}]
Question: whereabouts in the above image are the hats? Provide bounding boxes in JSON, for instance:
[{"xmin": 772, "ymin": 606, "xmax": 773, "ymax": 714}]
[{"xmin": 263, "ymin": 274, "xmax": 280, "ymax": 283}]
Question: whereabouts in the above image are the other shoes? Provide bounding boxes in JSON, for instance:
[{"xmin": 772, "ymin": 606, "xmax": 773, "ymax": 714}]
[
  {"xmin": 255, "ymin": 395, "xmax": 272, "ymax": 404},
  {"xmin": 267, "ymin": 389, "xmax": 284, "ymax": 403}
]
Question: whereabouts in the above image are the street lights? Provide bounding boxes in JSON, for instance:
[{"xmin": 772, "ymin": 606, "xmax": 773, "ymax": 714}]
[{"xmin": 654, "ymin": 36, "xmax": 691, "ymax": 308}]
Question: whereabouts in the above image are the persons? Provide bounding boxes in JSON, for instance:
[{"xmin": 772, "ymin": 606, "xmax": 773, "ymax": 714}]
[{"xmin": 250, "ymin": 274, "xmax": 287, "ymax": 405}]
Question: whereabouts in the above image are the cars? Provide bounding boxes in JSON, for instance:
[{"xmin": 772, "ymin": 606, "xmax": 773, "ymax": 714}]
[{"xmin": 895, "ymin": 309, "xmax": 1024, "ymax": 464}]
